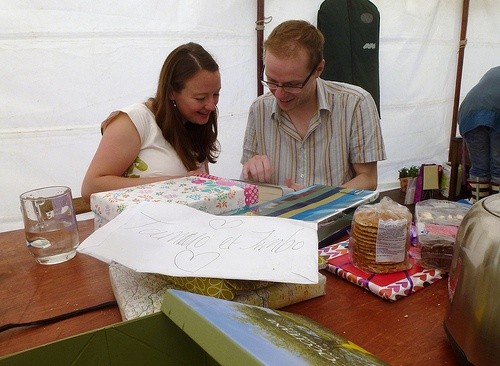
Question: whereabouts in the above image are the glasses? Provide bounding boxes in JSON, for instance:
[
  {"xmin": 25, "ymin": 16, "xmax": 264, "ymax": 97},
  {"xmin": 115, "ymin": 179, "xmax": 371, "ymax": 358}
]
[{"xmin": 259, "ymin": 63, "xmax": 321, "ymax": 92}]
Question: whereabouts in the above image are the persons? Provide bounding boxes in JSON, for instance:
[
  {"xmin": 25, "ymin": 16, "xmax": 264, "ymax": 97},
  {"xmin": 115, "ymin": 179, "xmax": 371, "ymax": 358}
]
[
  {"xmin": 458, "ymin": 66, "xmax": 499, "ymax": 208},
  {"xmin": 81, "ymin": 42, "xmax": 222, "ymax": 205},
  {"xmin": 239, "ymin": 20, "xmax": 387, "ymax": 193}
]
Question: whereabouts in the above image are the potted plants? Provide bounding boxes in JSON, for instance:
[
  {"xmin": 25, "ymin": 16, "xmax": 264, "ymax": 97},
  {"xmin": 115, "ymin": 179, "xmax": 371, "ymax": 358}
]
[{"xmin": 398, "ymin": 165, "xmax": 419, "ymax": 191}]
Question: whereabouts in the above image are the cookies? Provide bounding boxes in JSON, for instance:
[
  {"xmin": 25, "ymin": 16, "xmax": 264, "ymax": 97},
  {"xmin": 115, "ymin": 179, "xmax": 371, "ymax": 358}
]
[{"xmin": 349, "ymin": 210, "xmax": 413, "ymax": 274}]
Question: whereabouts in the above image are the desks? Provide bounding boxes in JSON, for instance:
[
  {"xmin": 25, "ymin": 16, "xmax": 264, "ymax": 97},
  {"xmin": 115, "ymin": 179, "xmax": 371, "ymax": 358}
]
[{"xmin": 0, "ymin": 218, "xmax": 468, "ymax": 366}]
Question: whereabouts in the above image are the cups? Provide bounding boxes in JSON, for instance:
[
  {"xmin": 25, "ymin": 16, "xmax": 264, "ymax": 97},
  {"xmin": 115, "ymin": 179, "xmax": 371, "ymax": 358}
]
[{"xmin": 20, "ymin": 186, "xmax": 79, "ymax": 264}]
